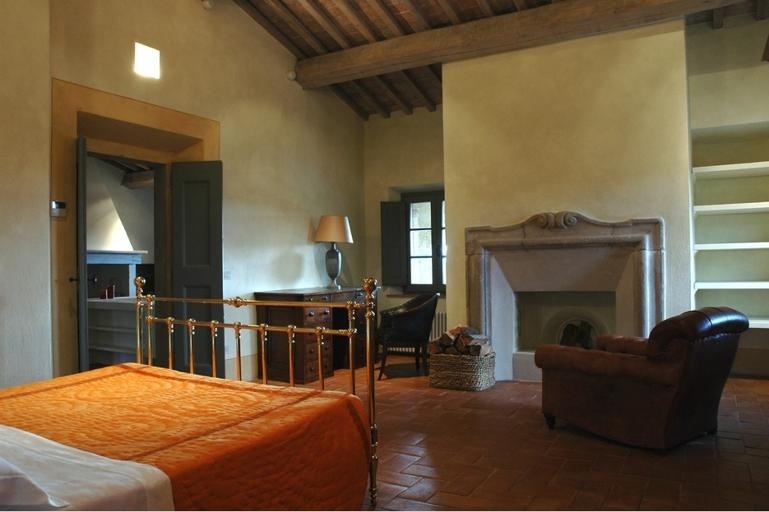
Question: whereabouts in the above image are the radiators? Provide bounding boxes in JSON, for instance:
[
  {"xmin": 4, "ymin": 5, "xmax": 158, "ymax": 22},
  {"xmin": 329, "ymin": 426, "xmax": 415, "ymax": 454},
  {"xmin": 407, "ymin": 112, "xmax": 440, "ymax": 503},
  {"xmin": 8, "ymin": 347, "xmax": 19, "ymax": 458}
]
[{"xmin": 377, "ymin": 311, "xmax": 446, "ymax": 355}]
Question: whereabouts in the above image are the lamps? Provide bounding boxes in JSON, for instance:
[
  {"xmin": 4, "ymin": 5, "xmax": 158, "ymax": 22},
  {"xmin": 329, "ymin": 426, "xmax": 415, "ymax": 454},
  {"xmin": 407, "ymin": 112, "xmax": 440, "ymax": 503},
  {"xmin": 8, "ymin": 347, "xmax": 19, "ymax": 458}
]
[{"xmin": 313, "ymin": 214, "xmax": 354, "ymax": 289}]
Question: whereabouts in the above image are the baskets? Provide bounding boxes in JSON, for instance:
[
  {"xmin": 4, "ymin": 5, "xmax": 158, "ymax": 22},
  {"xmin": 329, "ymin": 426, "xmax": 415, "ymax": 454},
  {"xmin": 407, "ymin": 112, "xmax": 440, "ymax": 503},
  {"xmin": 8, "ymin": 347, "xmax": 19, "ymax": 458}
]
[{"xmin": 428, "ymin": 352, "xmax": 496, "ymax": 391}]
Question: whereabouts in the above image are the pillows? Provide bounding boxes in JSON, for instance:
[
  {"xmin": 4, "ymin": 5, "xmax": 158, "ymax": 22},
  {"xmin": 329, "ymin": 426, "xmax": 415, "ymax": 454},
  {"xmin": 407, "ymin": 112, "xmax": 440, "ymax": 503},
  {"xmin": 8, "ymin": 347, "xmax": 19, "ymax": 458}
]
[{"xmin": 0, "ymin": 456, "xmax": 69, "ymax": 511}]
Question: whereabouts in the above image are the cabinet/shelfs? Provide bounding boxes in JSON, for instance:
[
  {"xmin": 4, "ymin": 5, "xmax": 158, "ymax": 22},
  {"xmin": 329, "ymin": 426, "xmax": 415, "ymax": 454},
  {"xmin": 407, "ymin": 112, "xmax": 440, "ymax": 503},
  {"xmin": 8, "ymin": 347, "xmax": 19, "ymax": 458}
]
[{"xmin": 688, "ymin": 64, "xmax": 769, "ymax": 333}]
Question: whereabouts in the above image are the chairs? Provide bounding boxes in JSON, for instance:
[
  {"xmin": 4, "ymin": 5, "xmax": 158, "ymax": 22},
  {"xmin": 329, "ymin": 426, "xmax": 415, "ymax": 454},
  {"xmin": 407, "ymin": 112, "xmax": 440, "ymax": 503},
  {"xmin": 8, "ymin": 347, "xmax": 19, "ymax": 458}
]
[
  {"xmin": 375, "ymin": 292, "xmax": 441, "ymax": 381},
  {"xmin": 534, "ymin": 305, "xmax": 751, "ymax": 455}
]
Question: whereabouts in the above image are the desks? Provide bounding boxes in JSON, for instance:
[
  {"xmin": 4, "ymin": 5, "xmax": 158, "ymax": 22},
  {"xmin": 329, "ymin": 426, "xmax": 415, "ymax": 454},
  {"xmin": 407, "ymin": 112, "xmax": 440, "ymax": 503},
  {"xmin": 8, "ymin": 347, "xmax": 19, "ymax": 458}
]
[{"xmin": 254, "ymin": 286, "xmax": 381, "ymax": 385}]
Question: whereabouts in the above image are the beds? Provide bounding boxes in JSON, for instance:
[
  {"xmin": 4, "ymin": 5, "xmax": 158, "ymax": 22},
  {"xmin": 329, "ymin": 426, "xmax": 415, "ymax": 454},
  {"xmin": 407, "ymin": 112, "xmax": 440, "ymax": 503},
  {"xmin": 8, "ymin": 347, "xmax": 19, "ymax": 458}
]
[{"xmin": 0, "ymin": 276, "xmax": 380, "ymax": 511}]
